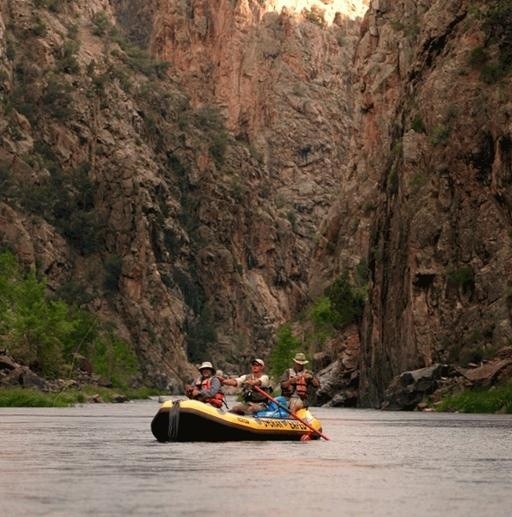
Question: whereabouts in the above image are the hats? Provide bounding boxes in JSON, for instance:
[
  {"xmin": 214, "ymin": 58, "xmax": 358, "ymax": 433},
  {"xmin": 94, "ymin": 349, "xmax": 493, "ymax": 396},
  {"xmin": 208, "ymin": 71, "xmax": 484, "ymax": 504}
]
[
  {"xmin": 199, "ymin": 362, "xmax": 214, "ymax": 369},
  {"xmin": 293, "ymin": 352, "xmax": 308, "ymax": 365},
  {"xmin": 252, "ymin": 358, "xmax": 264, "ymax": 367}
]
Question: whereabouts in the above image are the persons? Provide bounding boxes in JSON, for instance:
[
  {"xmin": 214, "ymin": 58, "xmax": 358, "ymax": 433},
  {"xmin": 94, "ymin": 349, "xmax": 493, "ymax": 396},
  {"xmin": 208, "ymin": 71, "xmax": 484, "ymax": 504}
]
[
  {"xmin": 184, "ymin": 362, "xmax": 224, "ymax": 407},
  {"xmin": 217, "ymin": 359, "xmax": 271, "ymax": 415},
  {"xmin": 274, "ymin": 353, "xmax": 320, "ymax": 419}
]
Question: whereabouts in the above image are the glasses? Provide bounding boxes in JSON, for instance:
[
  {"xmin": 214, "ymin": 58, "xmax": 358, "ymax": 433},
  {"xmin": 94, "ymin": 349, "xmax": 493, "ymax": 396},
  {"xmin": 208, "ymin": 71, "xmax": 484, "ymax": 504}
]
[{"xmin": 253, "ymin": 361, "xmax": 260, "ymax": 366}]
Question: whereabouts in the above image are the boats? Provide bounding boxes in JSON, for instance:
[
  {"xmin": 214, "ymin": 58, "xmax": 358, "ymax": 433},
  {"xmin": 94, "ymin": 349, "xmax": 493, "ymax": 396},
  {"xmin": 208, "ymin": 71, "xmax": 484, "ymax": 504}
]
[{"xmin": 151, "ymin": 396, "xmax": 323, "ymax": 441}]
